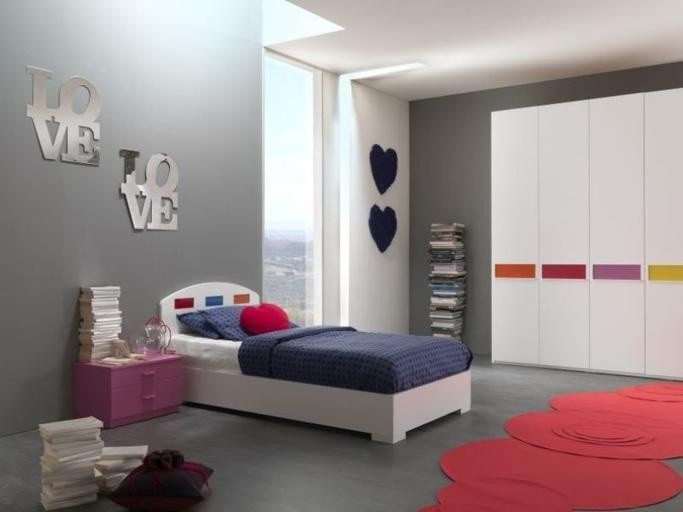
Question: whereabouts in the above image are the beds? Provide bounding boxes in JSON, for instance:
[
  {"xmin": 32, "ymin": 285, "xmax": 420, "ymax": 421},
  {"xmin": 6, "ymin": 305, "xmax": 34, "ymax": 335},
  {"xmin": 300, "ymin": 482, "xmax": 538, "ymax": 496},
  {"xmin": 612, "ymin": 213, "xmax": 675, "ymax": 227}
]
[{"xmin": 158, "ymin": 281, "xmax": 472, "ymax": 445}]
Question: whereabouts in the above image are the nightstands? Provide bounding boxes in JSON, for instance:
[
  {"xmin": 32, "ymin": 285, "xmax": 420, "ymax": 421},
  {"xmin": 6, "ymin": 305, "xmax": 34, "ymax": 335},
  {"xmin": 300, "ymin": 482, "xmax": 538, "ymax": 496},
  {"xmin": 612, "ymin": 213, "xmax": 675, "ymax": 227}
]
[{"xmin": 71, "ymin": 349, "xmax": 182, "ymax": 429}]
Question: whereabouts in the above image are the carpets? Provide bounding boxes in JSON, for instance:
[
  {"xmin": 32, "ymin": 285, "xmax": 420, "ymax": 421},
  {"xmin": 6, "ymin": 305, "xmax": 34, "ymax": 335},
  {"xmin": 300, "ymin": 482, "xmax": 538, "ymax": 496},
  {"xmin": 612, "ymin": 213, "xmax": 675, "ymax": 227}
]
[
  {"xmin": 436, "ymin": 475, "xmax": 571, "ymax": 512},
  {"xmin": 548, "ymin": 392, "xmax": 682, "ymax": 424},
  {"xmin": 620, "ymin": 378, "xmax": 683, "ymax": 402},
  {"xmin": 503, "ymin": 410, "xmax": 682, "ymax": 461},
  {"xmin": 438, "ymin": 436, "xmax": 682, "ymax": 511}
]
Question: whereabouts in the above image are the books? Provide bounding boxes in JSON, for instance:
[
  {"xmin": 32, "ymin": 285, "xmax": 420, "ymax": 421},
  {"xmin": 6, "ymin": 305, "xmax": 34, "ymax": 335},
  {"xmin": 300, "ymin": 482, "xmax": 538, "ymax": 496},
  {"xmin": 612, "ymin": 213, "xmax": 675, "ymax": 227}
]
[
  {"xmin": 100, "ymin": 340, "xmax": 145, "ymax": 367},
  {"xmin": 427, "ymin": 221, "xmax": 467, "ymax": 341},
  {"xmin": 76, "ymin": 285, "xmax": 121, "ymax": 364},
  {"xmin": 100, "ymin": 445, "xmax": 149, "ymax": 496},
  {"xmin": 38, "ymin": 416, "xmax": 105, "ymax": 510}
]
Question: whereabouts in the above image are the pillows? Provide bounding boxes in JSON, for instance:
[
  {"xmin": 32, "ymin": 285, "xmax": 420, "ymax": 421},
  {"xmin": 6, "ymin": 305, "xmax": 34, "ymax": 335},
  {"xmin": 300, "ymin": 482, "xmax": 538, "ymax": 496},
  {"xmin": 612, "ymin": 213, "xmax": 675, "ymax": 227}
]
[
  {"xmin": 175, "ymin": 312, "xmax": 222, "ymax": 339},
  {"xmin": 240, "ymin": 302, "xmax": 288, "ymax": 335},
  {"xmin": 198, "ymin": 304, "xmax": 299, "ymax": 342}
]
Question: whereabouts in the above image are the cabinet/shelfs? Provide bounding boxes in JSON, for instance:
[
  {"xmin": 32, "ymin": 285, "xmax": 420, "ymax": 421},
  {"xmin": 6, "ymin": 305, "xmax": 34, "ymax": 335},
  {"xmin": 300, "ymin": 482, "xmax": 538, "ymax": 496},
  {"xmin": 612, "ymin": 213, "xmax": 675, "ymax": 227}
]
[{"xmin": 488, "ymin": 85, "xmax": 682, "ymax": 383}]
